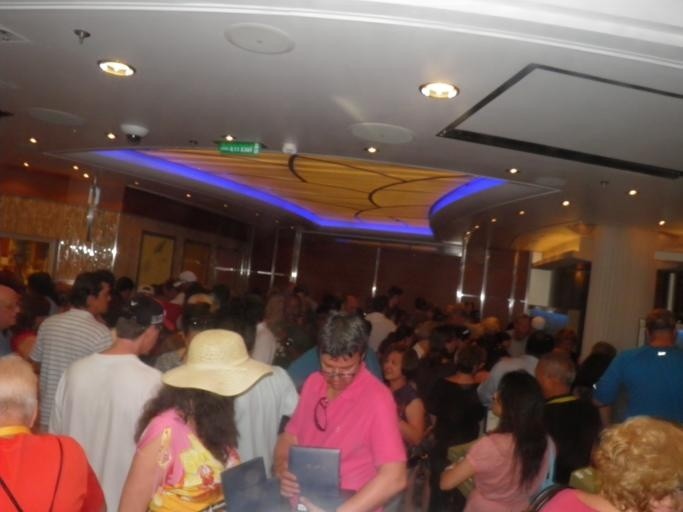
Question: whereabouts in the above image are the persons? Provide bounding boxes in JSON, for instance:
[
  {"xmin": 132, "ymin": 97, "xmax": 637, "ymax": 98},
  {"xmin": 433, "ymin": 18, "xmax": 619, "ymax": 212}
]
[{"xmin": 0, "ymin": 269, "xmax": 683, "ymax": 512}]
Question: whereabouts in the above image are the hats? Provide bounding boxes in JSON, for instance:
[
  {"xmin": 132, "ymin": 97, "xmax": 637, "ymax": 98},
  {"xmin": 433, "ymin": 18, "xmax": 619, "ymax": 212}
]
[
  {"xmin": 173, "ymin": 270, "xmax": 198, "ymax": 288},
  {"xmin": 162, "ymin": 328, "xmax": 273, "ymax": 399},
  {"xmin": 137, "ymin": 283, "xmax": 154, "ymax": 295}
]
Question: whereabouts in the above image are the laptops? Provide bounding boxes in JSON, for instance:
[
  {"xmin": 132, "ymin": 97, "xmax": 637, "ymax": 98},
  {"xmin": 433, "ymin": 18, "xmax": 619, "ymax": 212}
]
[
  {"xmin": 220, "ymin": 455, "xmax": 284, "ymax": 512},
  {"xmin": 289, "ymin": 444, "xmax": 356, "ymax": 512}
]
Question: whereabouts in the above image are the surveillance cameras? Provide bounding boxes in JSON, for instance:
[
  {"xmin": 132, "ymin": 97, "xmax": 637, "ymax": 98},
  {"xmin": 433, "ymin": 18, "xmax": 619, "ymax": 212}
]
[{"xmin": 122, "ymin": 124, "xmax": 150, "ymax": 143}]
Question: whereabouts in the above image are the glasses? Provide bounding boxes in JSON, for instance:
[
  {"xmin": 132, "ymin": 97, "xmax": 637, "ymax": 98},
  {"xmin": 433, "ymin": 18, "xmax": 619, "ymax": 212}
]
[{"xmin": 317, "ymin": 368, "xmax": 355, "ymax": 379}]
